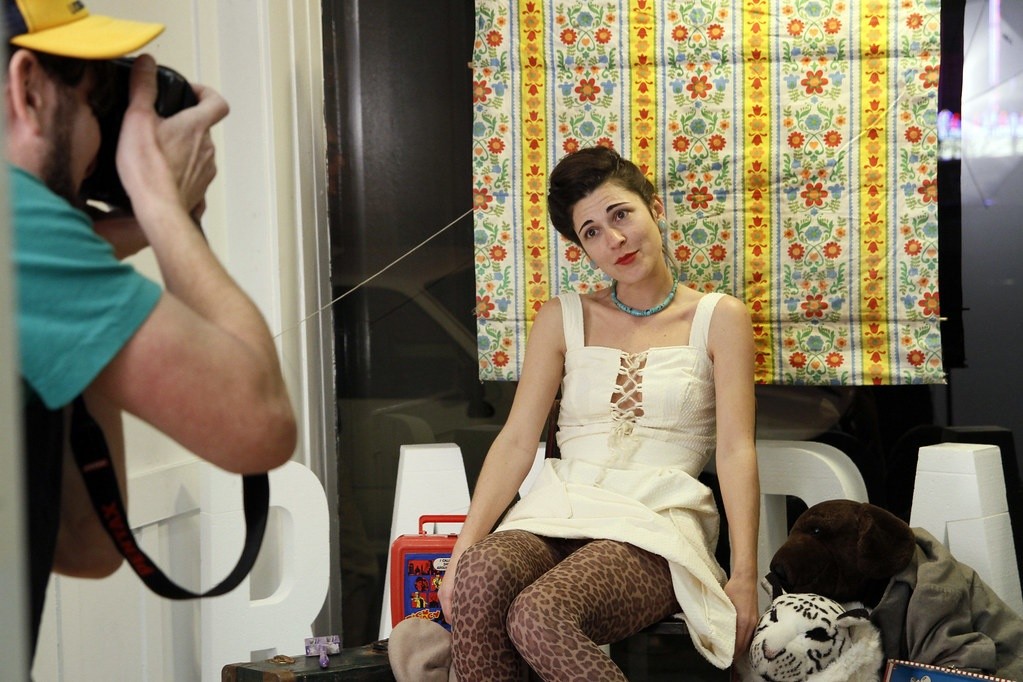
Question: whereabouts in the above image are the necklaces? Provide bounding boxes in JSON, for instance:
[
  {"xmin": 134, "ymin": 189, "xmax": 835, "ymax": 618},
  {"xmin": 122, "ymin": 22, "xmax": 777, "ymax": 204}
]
[{"xmin": 612, "ymin": 279, "xmax": 678, "ymax": 317}]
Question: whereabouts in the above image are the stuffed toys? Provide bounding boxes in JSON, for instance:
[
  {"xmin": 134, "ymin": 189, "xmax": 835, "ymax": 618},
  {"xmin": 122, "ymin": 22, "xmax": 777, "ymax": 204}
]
[
  {"xmin": 739, "ymin": 584, "xmax": 883, "ymax": 682},
  {"xmin": 769, "ymin": 498, "xmax": 1023, "ymax": 682}
]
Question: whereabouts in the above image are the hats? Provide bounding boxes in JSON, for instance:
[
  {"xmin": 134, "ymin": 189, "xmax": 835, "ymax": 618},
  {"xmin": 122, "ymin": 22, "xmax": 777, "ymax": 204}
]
[{"xmin": 1, "ymin": 0, "xmax": 164, "ymax": 60}]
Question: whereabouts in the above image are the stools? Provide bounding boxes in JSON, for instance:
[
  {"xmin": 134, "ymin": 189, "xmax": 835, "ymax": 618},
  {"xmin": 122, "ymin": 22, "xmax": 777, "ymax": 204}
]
[{"xmin": 611, "ymin": 621, "xmax": 732, "ymax": 682}]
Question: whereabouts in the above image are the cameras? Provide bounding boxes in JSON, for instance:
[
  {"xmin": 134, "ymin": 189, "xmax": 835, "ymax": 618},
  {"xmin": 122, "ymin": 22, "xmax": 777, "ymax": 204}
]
[{"xmin": 80, "ymin": 55, "xmax": 199, "ymax": 208}]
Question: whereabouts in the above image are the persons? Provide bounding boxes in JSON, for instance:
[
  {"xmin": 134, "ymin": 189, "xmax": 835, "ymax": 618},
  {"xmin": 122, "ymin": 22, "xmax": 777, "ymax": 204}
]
[
  {"xmin": 438, "ymin": 146, "xmax": 760, "ymax": 682},
  {"xmin": 0, "ymin": 0, "xmax": 299, "ymax": 682}
]
